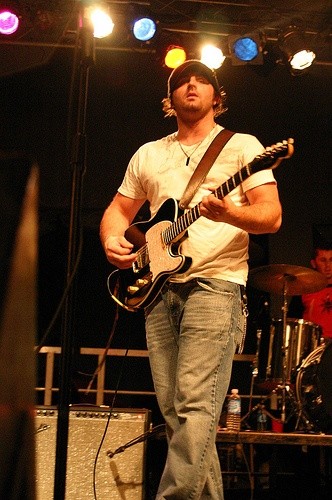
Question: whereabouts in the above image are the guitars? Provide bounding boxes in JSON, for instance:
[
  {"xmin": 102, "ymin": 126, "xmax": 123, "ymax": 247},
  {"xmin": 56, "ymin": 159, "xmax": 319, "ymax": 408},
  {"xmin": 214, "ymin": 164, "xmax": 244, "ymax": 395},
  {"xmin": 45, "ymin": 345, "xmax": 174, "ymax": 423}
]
[{"xmin": 114, "ymin": 138, "xmax": 295, "ymax": 313}]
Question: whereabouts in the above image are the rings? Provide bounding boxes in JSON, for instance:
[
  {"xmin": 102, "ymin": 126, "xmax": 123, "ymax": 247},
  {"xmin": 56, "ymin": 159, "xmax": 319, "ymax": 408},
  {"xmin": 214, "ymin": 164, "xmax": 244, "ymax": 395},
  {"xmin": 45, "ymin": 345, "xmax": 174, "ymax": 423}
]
[{"xmin": 216, "ymin": 212, "xmax": 220, "ymax": 215}]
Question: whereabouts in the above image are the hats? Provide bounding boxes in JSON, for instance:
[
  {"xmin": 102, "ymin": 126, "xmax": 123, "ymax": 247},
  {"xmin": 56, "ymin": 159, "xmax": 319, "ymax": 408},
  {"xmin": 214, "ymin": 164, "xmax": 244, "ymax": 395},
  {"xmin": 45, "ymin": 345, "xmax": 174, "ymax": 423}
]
[{"xmin": 167, "ymin": 58, "xmax": 221, "ymax": 98}]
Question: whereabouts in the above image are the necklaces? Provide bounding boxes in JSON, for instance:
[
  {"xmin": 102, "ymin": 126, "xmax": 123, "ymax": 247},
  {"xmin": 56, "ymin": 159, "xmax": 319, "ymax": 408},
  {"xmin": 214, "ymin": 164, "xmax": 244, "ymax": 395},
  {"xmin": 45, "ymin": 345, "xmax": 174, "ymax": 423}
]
[{"xmin": 177, "ymin": 135, "xmax": 209, "ymax": 166}]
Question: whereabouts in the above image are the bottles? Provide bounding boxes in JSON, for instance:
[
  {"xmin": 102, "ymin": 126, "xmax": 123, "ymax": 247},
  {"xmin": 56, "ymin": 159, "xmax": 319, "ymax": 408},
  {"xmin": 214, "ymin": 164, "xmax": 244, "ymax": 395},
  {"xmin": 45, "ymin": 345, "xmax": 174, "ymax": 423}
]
[
  {"xmin": 257, "ymin": 405, "xmax": 267, "ymax": 432},
  {"xmin": 226, "ymin": 389, "xmax": 241, "ymax": 433}
]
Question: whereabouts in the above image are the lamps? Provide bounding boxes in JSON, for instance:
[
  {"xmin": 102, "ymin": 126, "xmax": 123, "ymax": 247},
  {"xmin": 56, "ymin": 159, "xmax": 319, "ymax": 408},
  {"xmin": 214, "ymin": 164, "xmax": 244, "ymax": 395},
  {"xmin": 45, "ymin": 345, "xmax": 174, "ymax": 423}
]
[{"xmin": 0, "ymin": 0, "xmax": 316, "ymax": 77}]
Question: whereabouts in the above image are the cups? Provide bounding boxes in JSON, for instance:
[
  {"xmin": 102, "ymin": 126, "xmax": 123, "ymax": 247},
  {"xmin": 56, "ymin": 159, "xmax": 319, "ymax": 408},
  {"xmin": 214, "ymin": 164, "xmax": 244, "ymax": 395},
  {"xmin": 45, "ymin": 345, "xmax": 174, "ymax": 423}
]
[{"xmin": 272, "ymin": 419, "xmax": 283, "ymax": 433}]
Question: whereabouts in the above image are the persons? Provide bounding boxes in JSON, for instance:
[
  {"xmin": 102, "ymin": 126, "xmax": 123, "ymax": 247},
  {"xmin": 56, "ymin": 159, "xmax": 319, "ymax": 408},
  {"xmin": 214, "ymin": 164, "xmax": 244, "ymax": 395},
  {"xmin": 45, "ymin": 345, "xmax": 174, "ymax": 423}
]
[
  {"xmin": 100, "ymin": 59, "xmax": 282, "ymax": 500},
  {"xmin": 300, "ymin": 238, "xmax": 332, "ymax": 340}
]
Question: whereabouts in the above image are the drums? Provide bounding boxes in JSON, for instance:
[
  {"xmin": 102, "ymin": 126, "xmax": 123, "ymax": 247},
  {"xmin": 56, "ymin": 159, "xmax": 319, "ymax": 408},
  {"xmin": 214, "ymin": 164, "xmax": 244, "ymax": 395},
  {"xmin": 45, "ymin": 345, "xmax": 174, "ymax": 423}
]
[
  {"xmin": 291, "ymin": 339, "xmax": 332, "ymax": 433},
  {"xmin": 256, "ymin": 318, "xmax": 322, "ymax": 390}
]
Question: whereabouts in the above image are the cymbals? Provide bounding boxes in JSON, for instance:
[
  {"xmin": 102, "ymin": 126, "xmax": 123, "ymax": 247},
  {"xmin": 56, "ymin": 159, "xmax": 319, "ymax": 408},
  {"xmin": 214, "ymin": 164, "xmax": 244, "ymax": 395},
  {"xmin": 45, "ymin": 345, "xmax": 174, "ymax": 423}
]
[{"xmin": 244, "ymin": 263, "xmax": 326, "ymax": 297}]
